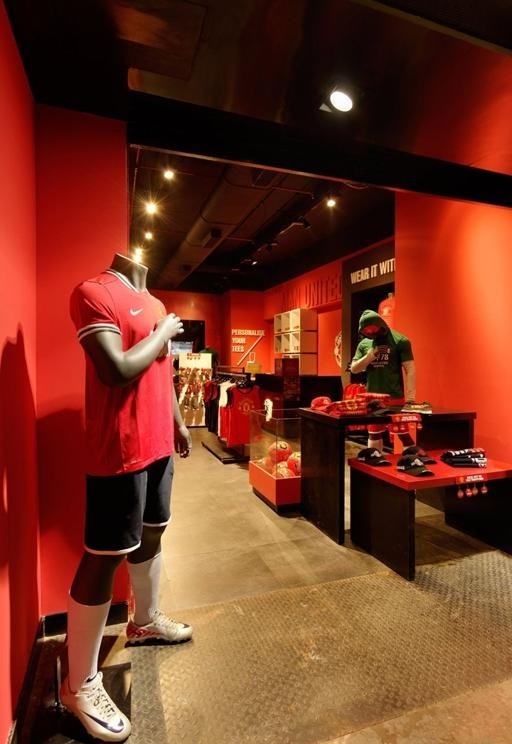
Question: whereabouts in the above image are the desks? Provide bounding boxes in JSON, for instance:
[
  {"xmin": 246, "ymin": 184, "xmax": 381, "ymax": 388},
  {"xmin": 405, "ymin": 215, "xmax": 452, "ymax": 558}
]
[{"xmin": 298, "ymin": 406, "xmax": 512, "ymax": 581}]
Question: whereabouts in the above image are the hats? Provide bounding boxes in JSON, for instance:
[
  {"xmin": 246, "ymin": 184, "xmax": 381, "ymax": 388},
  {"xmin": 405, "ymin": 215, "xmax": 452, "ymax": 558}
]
[
  {"xmin": 396, "ymin": 446, "xmax": 435, "ymax": 477},
  {"xmin": 311, "ymin": 397, "xmax": 333, "ymax": 411},
  {"xmin": 359, "ymin": 447, "xmax": 392, "ymax": 465}
]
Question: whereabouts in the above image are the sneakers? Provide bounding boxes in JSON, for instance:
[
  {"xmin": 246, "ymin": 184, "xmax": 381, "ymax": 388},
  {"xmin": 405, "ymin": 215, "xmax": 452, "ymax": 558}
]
[
  {"xmin": 60, "ymin": 670, "xmax": 132, "ymax": 742},
  {"xmin": 127, "ymin": 609, "xmax": 193, "ymax": 644}
]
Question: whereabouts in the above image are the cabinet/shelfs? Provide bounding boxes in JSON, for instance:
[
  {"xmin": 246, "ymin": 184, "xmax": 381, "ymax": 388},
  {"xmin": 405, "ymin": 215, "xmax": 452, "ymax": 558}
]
[{"xmin": 273, "ymin": 308, "xmax": 318, "ymax": 375}]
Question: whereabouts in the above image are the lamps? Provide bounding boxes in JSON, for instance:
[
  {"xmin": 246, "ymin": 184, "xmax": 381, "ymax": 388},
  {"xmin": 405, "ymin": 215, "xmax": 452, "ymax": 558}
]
[
  {"xmin": 300, "ymin": 216, "xmax": 312, "ymax": 231},
  {"xmin": 318, "ymin": 67, "xmax": 367, "ymax": 114}
]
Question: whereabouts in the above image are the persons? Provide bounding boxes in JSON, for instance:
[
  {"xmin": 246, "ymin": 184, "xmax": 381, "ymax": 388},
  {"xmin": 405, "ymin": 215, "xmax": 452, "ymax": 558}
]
[
  {"xmin": 54, "ymin": 250, "xmax": 197, "ymax": 741},
  {"xmin": 350, "ymin": 309, "xmax": 418, "ymax": 454},
  {"xmin": 378, "ymin": 292, "xmax": 394, "ymax": 330}
]
